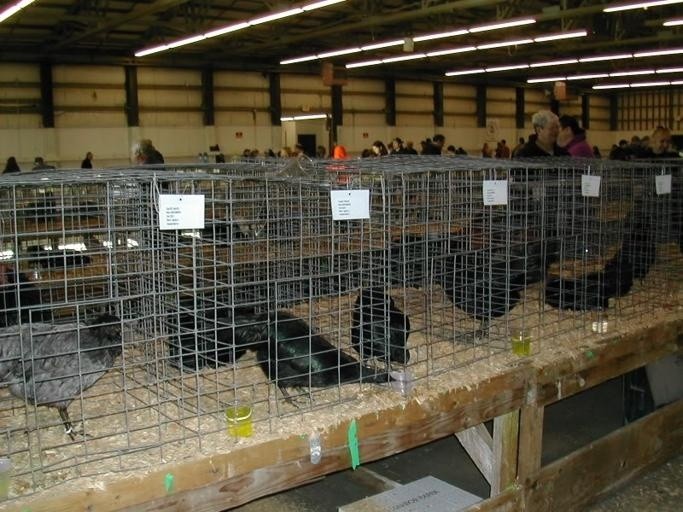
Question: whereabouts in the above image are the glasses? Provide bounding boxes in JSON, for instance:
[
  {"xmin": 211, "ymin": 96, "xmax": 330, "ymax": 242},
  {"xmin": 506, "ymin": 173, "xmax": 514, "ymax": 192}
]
[
  {"xmin": 1, "ymin": 0, "xmax": 36, "ymax": 22},
  {"xmin": 133, "ymin": 0, "xmax": 304, "ymax": 58}
]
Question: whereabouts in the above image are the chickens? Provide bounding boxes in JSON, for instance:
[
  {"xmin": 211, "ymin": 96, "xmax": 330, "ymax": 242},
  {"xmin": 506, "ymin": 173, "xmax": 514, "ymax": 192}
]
[
  {"xmin": 429, "ymin": 207, "xmax": 656, "ymax": 344},
  {"xmin": 18, "ymin": 191, "xmax": 105, "ymax": 226},
  {"xmin": 24, "ymin": 242, "xmax": 92, "ymax": 273},
  {"xmin": 0, "ymin": 267, "xmax": 125, "ymax": 444}
]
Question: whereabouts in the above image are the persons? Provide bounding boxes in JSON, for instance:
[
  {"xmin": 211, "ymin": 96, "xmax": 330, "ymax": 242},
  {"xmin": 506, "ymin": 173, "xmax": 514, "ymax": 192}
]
[
  {"xmin": 209, "ymin": 144, "xmax": 225, "ymax": 163},
  {"xmin": 32, "ymin": 156, "xmax": 55, "ymax": 170},
  {"xmin": 420, "ymin": 134, "xmax": 467, "ymax": 156},
  {"xmin": 362, "ymin": 137, "xmax": 418, "ymax": 158},
  {"xmin": 609, "ymin": 126, "xmax": 680, "ymax": 175},
  {"xmin": 241, "ymin": 142, "xmax": 313, "ymax": 173},
  {"xmin": 4, "ymin": 156, "xmax": 21, "ymax": 173},
  {"xmin": 316, "ymin": 145, "xmax": 328, "ymax": 165},
  {"xmin": 81, "ymin": 152, "xmax": 93, "ymax": 169},
  {"xmin": 511, "ymin": 110, "xmax": 602, "ymax": 182},
  {"xmin": 135, "ymin": 139, "xmax": 165, "ymax": 170},
  {"xmin": 483, "ymin": 139, "xmax": 510, "ymax": 159}
]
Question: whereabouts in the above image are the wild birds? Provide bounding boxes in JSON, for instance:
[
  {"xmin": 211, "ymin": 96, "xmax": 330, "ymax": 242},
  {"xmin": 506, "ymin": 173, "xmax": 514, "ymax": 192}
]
[
  {"xmin": 351, "ymin": 285, "xmax": 410, "ymax": 370},
  {"xmin": 168, "ymin": 213, "xmax": 417, "ymax": 307},
  {"xmin": 170, "ymin": 304, "xmax": 397, "ymax": 404}
]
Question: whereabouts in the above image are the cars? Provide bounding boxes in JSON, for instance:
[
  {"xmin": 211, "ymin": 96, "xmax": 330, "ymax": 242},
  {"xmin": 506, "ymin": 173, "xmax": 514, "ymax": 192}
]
[
  {"xmin": 224, "ymin": 405, "xmax": 253, "ymax": 441},
  {"xmin": 389, "ymin": 371, "xmax": 414, "ymax": 397},
  {"xmin": 591, "ymin": 308, "xmax": 609, "ymax": 336},
  {"xmin": 510, "ymin": 328, "xmax": 532, "ymax": 358}
]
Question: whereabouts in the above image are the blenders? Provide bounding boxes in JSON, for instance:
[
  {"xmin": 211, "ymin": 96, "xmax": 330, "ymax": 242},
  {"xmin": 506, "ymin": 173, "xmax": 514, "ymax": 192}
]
[
  {"xmin": 0, "ymin": 267, "xmax": 125, "ymax": 444},
  {"xmin": 429, "ymin": 207, "xmax": 656, "ymax": 344},
  {"xmin": 24, "ymin": 242, "xmax": 92, "ymax": 273},
  {"xmin": 18, "ymin": 191, "xmax": 105, "ymax": 226}
]
